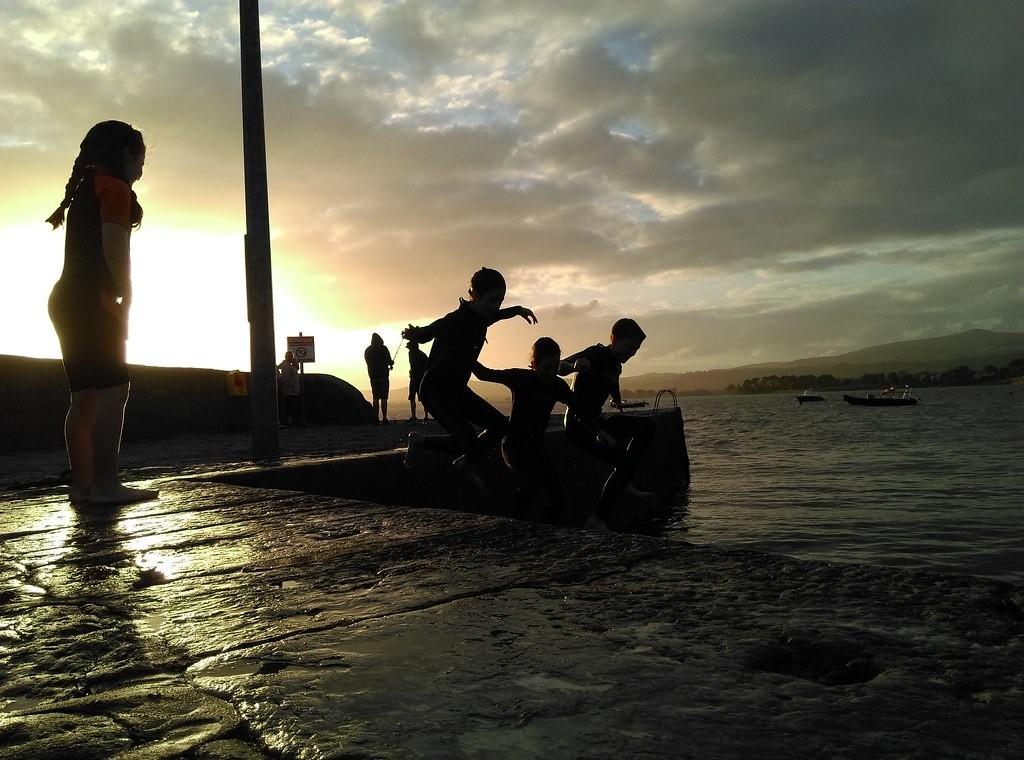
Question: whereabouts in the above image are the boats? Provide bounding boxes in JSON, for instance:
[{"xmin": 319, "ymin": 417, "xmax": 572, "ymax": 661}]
[
  {"xmin": 840, "ymin": 395, "xmax": 917, "ymax": 406},
  {"xmin": 610, "ymin": 401, "xmax": 646, "ymax": 409},
  {"xmin": 795, "ymin": 390, "xmax": 824, "ymax": 403}
]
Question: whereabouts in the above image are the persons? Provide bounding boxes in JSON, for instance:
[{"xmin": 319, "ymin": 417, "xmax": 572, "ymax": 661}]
[
  {"xmin": 364, "ymin": 332, "xmax": 394, "ymax": 426},
  {"xmin": 277, "ymin": 351, "xmax": 308, "ymax": 430},
  {"xmin": 556, "ymin": 319, "xmax": 656, "ymax": 535},
  {"xmin": 458, "ymin": 336, "xmax": 619, "ymax": 525},
  {"xmin": 401, "ymin": 267, "xmax": 538, "ymax": 479},
  {"xmin": 44, "ymin": 121, "xmax": 162, "ymax": 505},
  {"xmin": 405, "ymin": 339, "xmax": 430, "ymax": 425}
]
